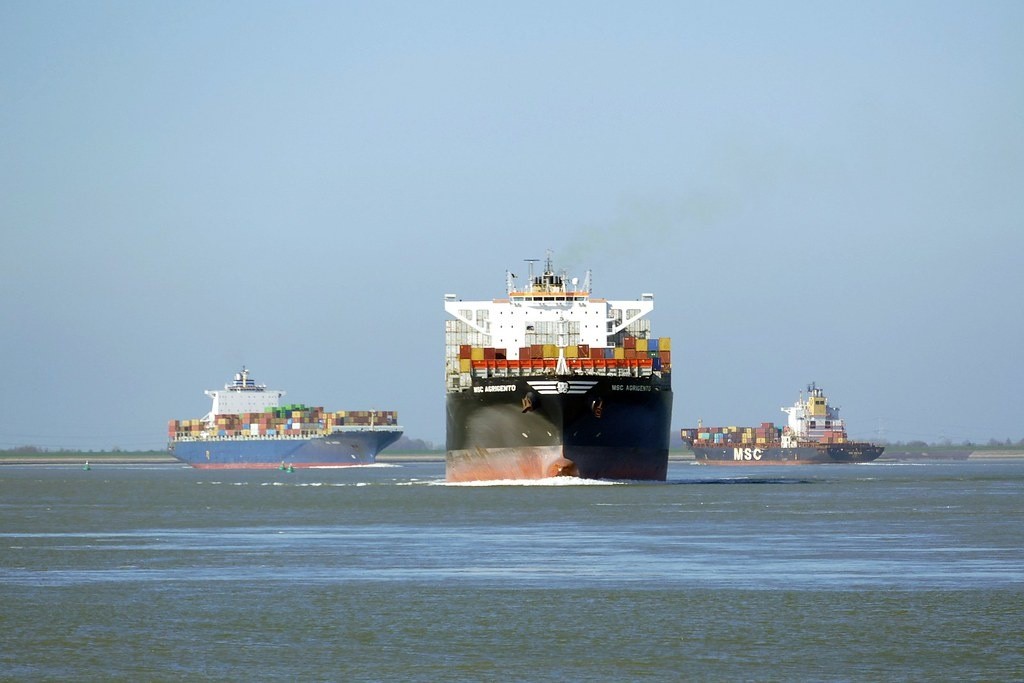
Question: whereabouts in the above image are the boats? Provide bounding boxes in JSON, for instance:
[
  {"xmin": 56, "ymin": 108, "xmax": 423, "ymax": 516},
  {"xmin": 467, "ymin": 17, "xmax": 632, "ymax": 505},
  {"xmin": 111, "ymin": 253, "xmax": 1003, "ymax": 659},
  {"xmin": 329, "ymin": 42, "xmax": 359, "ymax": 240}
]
[
  {"xmin": 681, "ymin": 381, "xmax": 885, "ymax": 464},
  {"xmin": 444, "ymin": 249, "xmax": 673, "ymax": 483},
  {"xmin": 168, "ymin": 370, "xmax": 404, "ymax": 470}
]
